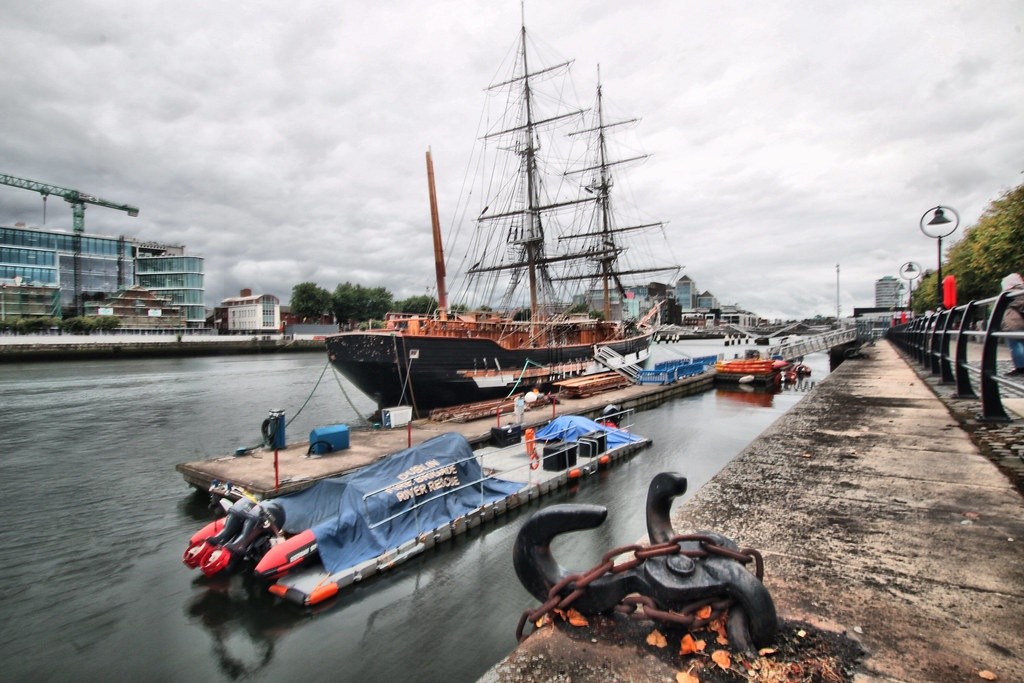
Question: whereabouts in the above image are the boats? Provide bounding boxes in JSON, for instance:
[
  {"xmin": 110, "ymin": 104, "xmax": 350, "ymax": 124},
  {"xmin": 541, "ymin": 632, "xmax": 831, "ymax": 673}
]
[{"xmin": 183, "ymin": 433, "xmax": 463, "ymax": 579}]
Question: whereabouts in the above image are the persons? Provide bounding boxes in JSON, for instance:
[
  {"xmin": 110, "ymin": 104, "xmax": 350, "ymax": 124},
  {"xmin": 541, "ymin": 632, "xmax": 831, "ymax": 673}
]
[{"xmin": 998, "ymin": 258, "xmax": 1023, "ymax": 376}]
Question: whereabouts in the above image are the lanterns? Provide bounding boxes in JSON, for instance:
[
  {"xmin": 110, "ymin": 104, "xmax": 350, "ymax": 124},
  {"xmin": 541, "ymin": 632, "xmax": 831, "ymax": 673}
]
[
  {"xmin": 943, "ymin": 274, "xmax": 957, "ymax": 310},
  {"xmin": 901, "ymin": 312, "xmax": 906, "ymax": 323}
]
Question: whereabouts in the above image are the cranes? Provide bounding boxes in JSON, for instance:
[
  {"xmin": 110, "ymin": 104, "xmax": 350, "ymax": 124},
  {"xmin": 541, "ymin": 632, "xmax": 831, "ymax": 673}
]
[{"xmin": 1, "ymin": 173, "xmax": 139, "ymax": 324}]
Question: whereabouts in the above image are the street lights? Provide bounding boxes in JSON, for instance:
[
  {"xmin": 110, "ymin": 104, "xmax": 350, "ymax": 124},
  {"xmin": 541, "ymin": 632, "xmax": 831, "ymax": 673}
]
[
  {"xmin": 899, "ymin": 262, "xmax": 920, "ymax": 320},
  {"xmin": 920, "ymin": 206, "xmax": 960, "ymax": 309}
]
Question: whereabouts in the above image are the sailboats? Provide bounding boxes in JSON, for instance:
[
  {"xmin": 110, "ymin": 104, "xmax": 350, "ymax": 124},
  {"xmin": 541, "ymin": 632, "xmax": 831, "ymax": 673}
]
[{"xmin": 326, "ymin": 3, "xmax": 659, "ymax": 416}]
